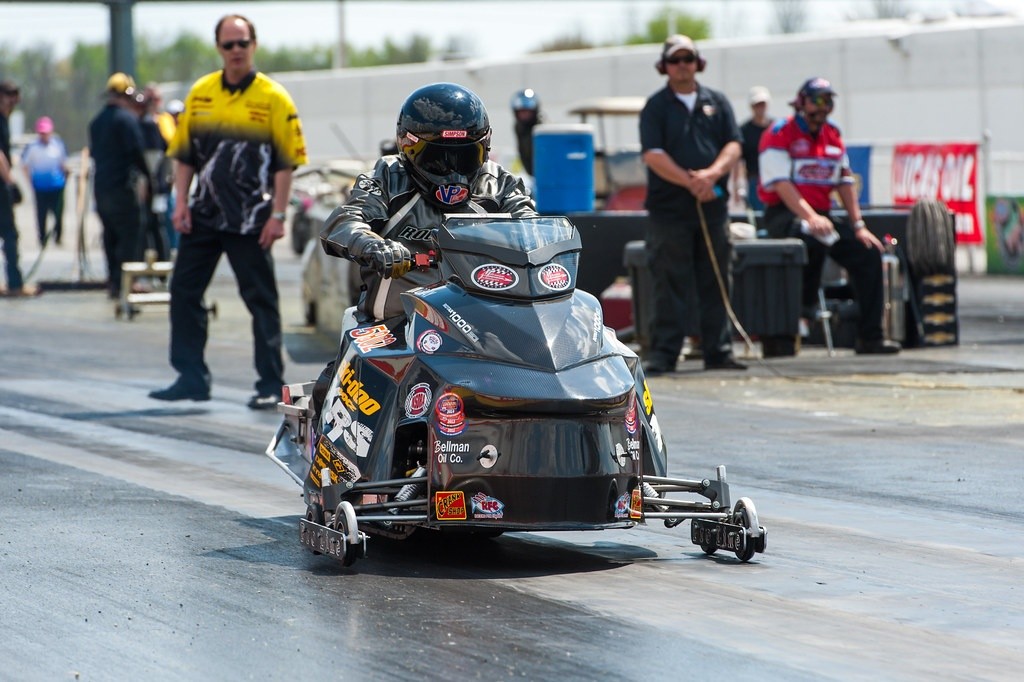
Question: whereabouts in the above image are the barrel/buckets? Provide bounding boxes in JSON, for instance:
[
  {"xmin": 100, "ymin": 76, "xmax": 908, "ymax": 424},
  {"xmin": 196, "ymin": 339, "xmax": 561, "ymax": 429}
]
[{"xmin": 533, "ymin": 123, "xmax": 596, "ymax": 215}]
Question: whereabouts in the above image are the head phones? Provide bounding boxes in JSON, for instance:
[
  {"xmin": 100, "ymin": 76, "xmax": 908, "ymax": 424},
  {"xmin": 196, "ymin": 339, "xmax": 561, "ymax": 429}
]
[{"xmin": 656, "ymin": 55, "xmax": 706, "ymax": 75}]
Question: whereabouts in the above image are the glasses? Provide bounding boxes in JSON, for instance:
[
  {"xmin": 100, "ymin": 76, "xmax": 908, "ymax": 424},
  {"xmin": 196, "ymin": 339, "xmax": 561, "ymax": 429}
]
[
  {"xmin": 218, "ymin": 38, "xmax": 253, "ymax": 50},
  {"xmin": 803, "ymin": 97, "xmax": 832, "ymax": 106},
  {"xmin": 668, "ymin": 54, "xmax": 697, "ymax": 66}
]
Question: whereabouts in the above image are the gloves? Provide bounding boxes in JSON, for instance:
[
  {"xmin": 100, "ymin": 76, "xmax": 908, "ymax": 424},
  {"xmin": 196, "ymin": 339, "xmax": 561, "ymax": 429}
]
[{"xmin": 349, "ymin": 232, "xmax": 410, "ymax": 280}]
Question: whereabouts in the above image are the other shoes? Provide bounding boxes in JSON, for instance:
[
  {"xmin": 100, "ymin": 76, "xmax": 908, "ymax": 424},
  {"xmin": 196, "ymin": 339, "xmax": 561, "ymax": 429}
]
[
  {"xmin": 148, "ymin": 379, "xmax": 211, "ymax": 402},
  {"xmin": 640, "ymin": 360, "xmax": 665, "ymax": 376},
  {"xmin": 703, "ymin": 353, "xmax": 748, "ymax": 371},
  {"xmin": 855, "ymin": 337, "xmax": 902, "ymax": 355},
  {"xmin": 115, "ymin": 305, "xmax": 139, "ymax": 317},
  {"xmin": 248, "ymin": 392, "xmax": 279, "ymax": 410}
]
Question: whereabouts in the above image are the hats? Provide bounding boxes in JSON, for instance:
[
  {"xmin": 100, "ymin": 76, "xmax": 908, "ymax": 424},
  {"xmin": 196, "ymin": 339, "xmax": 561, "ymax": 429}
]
[
  {"xmin": 749, "ymin": 86, "xmax": 769, "ymax": 106},
  {"xmin": 798, "ymin": 77, "xmax": 838, "ymax": 98},
  {"xmin": 662, "ymin": 34, "xmax": 697, "ymax": 58},
  {"xmin": 37, "ymin": 117, "xmax": 53, "ymax": 134}
]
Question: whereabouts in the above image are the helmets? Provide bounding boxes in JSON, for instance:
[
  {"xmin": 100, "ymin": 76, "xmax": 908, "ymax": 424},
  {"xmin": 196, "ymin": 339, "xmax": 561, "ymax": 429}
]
[
  {"xmin": 395, "ymin": 82, "xmax": 492, "ymax": 208},
  {"xmin": 509, "ymin": 89, "xmax": 538, "ymax": 123},
  {"xmin": 105, "ymin": 72, "xmax": 134, "ymax": 94}
]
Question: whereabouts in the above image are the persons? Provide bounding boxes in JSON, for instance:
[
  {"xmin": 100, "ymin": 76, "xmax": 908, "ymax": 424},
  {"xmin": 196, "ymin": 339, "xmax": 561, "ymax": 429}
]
[
  {"xmin": 737, "ymin": 87, "xmax": 782, "ymax": 204},
  {"xmin": 18, "ymin": 117, "xmax": 71, "ymax": 250},
  {"xmin": 146, "ymin": 14, "xmax": 308, "ymax": 410},
  {"xmin": 633, "ymin": 35, "xmax": 750, "ymax": 375},
  {"xmin": 86, "ymin": 72, "xmax": 186, "ymax": 321},
  {"xmin": 756, "ymin": 77, "xmax": 902, "ymax": 356},
  {"xmin": 504, "ymin": 87, "xmax": 544, "ymax": 174},
  {"xmin": 313, "ymin": 86, "xmax": 540, "ymax": 427},
  {"xmin": 0, "ymin": 78, "xmax": 40, "ymax": 297}
]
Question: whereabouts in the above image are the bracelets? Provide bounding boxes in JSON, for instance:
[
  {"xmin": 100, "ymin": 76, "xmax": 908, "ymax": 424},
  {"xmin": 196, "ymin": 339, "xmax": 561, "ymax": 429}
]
[
  {"xmin": 851, "ymin": 219, "xmax": 865, "ymax": 230},
  {"xmin": 270, "ymin": 211, "xmax": 286, "ymax": 222}
]
